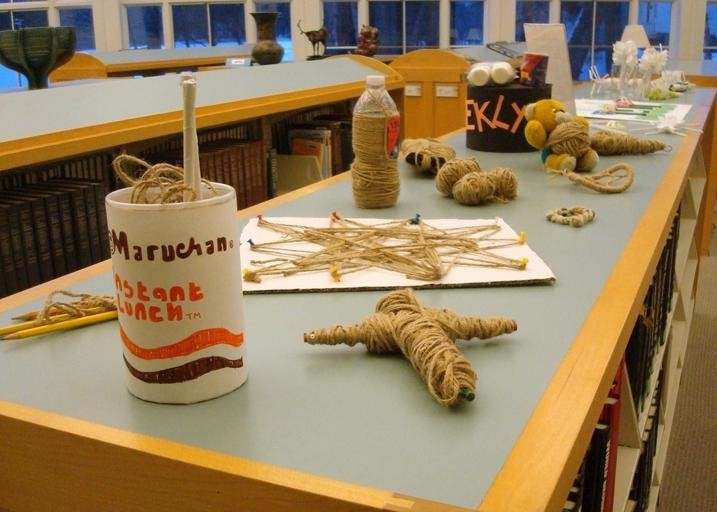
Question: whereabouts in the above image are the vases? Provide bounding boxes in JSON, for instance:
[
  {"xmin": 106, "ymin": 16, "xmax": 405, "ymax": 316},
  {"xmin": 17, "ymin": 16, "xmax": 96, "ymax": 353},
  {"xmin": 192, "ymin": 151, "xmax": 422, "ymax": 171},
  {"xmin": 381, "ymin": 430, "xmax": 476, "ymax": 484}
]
[{"xmin": 249, "ymin": 12, "xmax": 284, "ymax": 64}]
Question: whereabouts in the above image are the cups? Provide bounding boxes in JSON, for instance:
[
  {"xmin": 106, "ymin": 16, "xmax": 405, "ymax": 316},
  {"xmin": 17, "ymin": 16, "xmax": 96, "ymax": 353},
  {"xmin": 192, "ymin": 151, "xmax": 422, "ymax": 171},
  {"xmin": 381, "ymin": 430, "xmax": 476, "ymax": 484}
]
[{"xmin": 518, "ymin": 53, "xmax": 549, "ymax": 87}]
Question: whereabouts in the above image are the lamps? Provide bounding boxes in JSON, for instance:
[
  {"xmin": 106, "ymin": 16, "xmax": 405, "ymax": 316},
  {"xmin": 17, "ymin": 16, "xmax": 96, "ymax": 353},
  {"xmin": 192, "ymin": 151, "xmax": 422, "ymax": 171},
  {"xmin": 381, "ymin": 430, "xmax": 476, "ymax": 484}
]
[{"xmin": 621, "ymin": 25, "xmax": 650, "ymax": 101}]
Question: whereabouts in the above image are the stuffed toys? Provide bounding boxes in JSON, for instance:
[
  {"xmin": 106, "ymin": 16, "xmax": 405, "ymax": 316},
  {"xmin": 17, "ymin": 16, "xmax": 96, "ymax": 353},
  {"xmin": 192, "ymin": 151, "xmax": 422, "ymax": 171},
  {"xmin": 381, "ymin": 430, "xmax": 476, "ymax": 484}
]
[{"xmin": 523, "ymin": 97, "xmax": 598, "ymax": 170}]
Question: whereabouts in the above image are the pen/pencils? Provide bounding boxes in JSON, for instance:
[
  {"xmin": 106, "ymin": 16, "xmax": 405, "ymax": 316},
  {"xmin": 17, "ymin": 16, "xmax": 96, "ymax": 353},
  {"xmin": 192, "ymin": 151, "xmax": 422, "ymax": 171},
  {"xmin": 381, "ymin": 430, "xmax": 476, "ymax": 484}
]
[{"xmin": 0, "ymin": 305, "xmax": 118, "ymax": 340}]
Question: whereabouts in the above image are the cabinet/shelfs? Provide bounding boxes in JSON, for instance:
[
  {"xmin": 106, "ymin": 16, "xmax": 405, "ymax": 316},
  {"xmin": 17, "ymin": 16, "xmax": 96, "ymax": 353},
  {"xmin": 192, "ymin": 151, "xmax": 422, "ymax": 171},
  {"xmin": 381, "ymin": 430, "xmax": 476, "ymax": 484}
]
[{"xmin": 0, "ymin": 48, "xmax": 716, "ymax": 512}]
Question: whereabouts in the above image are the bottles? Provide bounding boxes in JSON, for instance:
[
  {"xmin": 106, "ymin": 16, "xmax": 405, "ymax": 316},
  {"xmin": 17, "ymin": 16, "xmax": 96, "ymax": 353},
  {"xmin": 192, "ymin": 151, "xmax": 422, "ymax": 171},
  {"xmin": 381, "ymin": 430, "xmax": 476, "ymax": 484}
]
[{"xmin": 348, "ymin": 73, "xmax": 399, "ymax": 210}]
